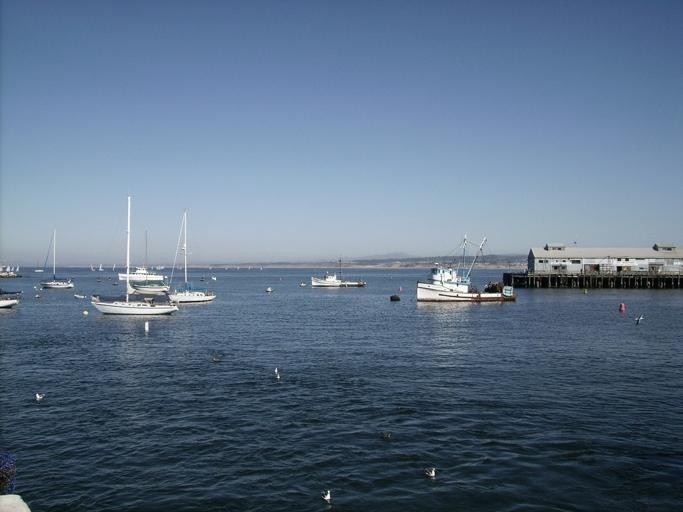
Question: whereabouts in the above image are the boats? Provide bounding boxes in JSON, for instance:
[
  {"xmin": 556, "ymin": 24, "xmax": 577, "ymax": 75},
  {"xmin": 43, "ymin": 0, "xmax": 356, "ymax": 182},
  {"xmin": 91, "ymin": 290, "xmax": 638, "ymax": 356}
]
[
  {"xmin": 39, "ymin": 228, "xmax": 74, "ymax": 289},
  {"xmin": 0, "ymin": 266, "xmax": 23, "ymax": 309},
  {"xmin": 90, "ymin": 194, "xmax": 218, "ymax": 316},
  {"xmin": 311, "ymin": 270, "xmax": 368, "ymax": 287},
  {"xmin": 415, "ymin": 231, "xmax": 518, "ymax": 301}
]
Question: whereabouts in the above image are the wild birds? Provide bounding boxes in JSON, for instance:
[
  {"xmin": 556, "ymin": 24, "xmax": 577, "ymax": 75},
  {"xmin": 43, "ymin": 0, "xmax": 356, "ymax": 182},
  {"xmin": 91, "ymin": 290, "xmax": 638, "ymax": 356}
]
[
  {"xmin": 635, "ymin": 313, "xmax": 645, "ymax": 327},
  {"xmin": 36, "ymin": 393, "xmax": 45, "ymax": 398},
  {"xmin": 274, "ymin": 368, "xmax": 280, "ymax": 380},
  {"xmin": 423, "ymin": 467, "xmax": 436, "ymax": 477},
  {"xmin": 320, "ymin": 490, "xmax": 331, "ymax": 500}
]
